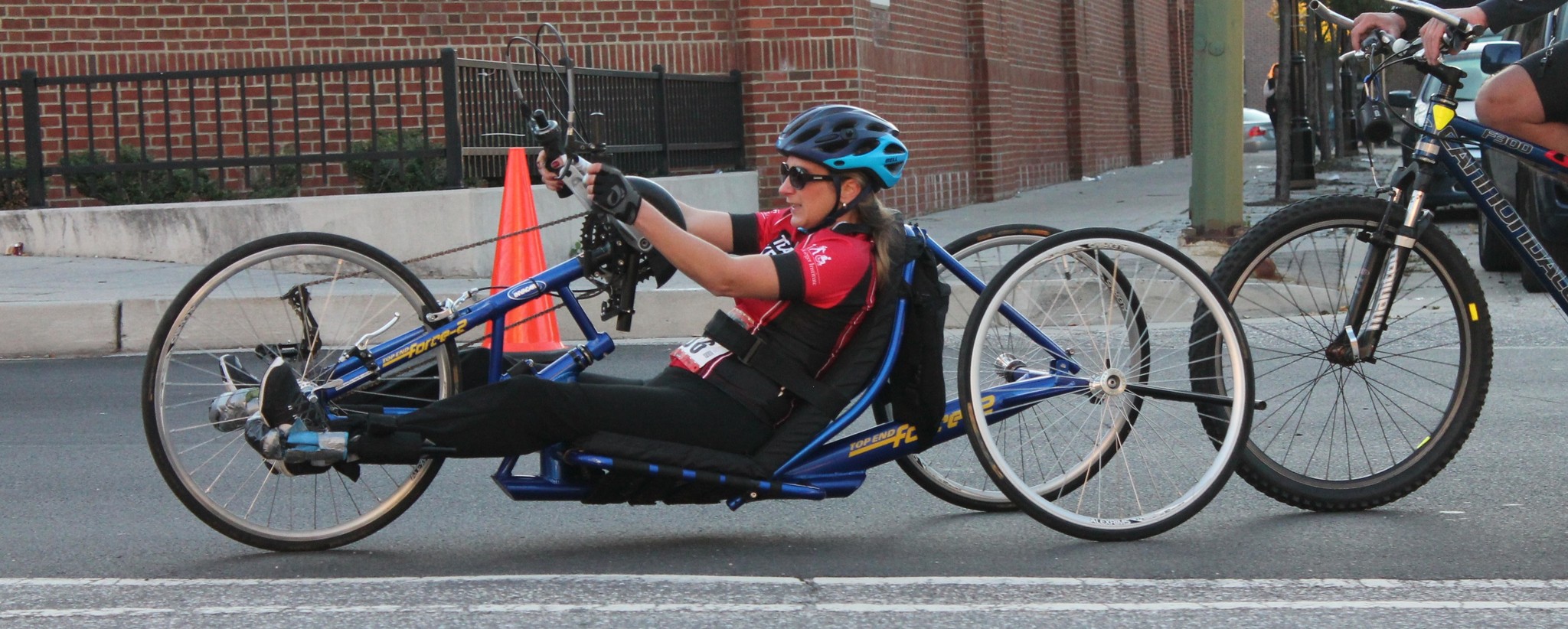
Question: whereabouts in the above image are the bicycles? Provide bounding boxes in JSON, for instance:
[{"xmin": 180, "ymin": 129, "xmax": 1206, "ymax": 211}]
[{"xmin": 1184, "ymin": 0, "xmax": 1568, "ymax": 513}]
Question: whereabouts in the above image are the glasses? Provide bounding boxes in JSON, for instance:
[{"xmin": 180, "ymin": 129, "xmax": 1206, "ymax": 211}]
[{"xmin": 781, "ymin": 162, "xmax": 833, "ymax": 189}]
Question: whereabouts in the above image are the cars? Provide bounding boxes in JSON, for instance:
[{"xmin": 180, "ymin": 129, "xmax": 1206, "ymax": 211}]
[
  {"xmin": 1242, "ymin": 106, "xmax": 1277, "ymax": 154},
  {"xmin": 1478, "ymin": 4, "xmax": 1568, "ymax": 294},
  {"xmin": 1389, "ymin": 40, "xmax": 1525, "ymax": 217}
]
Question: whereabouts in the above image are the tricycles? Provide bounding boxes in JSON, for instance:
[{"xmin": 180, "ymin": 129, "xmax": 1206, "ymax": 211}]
[{"xmin": 139, "ymin": 19, "xmax": 1268, "ymax": 557}]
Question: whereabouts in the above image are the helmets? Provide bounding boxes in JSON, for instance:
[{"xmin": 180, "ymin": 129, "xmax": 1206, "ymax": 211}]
[{"xmin": 776, "ymin": 104, "xmax": 910, "ymax": 193}]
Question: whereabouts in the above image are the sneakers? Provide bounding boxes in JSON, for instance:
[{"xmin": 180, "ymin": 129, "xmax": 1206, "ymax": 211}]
[
  {"xmin": 258, "ymin": 355, "xmax": 333, "ymax": 479},
  {"xmin": 220, "ymin": 354, "xmax": 283, "ymax": 476}
]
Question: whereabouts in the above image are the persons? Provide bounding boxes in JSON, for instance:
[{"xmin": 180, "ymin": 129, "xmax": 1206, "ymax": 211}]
[
  {"xmin": 1262, "ymin": 63, "xmax": 1281, "ymax": 131},
  {"xmin": 1351, "ymin": 0, "xmax": 1568, "ymax": 209},
  {"xmin": 218, "ymin": 104, "xmax": 911, "ymax": 483}
]
